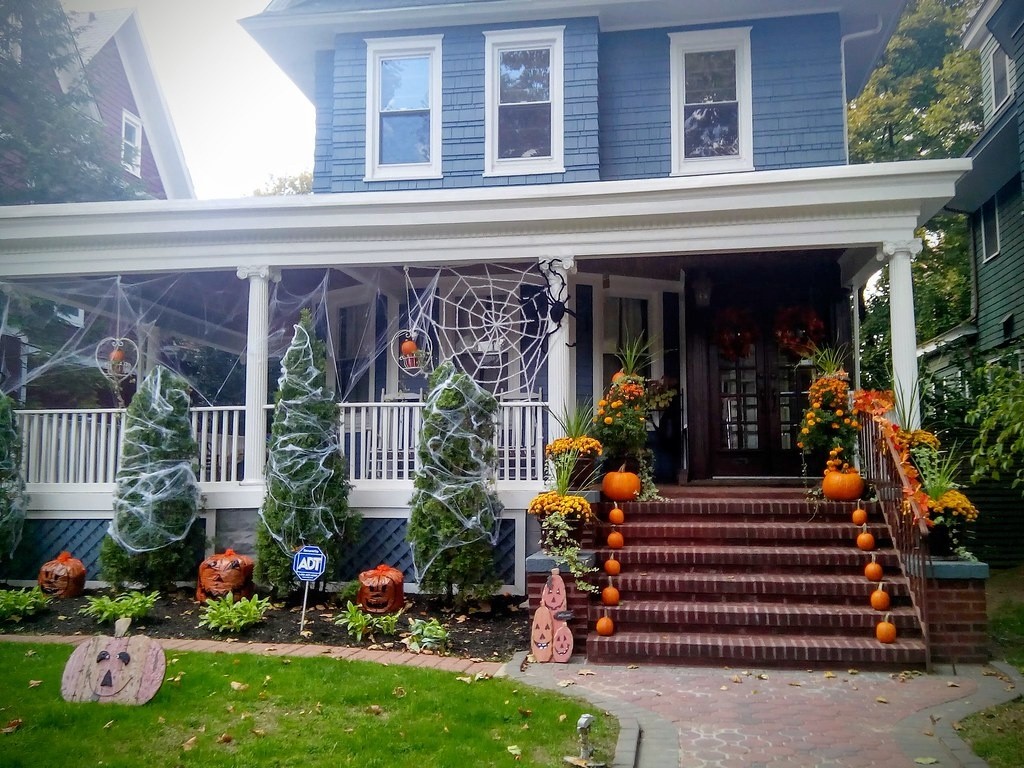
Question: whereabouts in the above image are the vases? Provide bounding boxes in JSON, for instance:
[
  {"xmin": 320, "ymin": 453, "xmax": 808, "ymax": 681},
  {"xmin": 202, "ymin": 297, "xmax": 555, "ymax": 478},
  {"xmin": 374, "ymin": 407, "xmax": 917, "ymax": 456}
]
[
  {"xmin": 885, "ymin": 451, "xmax": 903, "ymax": 482},
  {"xmin": 556, "ymin": 454, "xmax": 594, "ymax": 492},
  {"xmin": 536, "ymin": 517, "xmax": 586, "ymax": 554},
  {"xmin": 923, "ymin": 509, "xmax": 949, "ymax": 557}
]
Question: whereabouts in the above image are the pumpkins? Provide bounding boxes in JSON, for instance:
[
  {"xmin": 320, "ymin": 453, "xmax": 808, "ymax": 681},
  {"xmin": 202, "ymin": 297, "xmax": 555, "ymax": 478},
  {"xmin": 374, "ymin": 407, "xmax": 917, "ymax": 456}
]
[
  {"xmin": 853, "ymin": 498, "xmax": 896, "ymax": 643},
  {"xmin": 109, "ymin": 348, "xmax": 124, "ymax": 362},
  {"xmin": 822, "ymin": 467, "xmax": 863, "ymax": 501},
  {"xmin": 596, "ymin": 502, "xmax": 624, "ymax": 636},
  {"xmin": 602, "ymin": 463, "xmax": 640, "ymax": 502},
  {"xmin": 402, "ymin": 339, "xmax": 417, "ymax": 356}
]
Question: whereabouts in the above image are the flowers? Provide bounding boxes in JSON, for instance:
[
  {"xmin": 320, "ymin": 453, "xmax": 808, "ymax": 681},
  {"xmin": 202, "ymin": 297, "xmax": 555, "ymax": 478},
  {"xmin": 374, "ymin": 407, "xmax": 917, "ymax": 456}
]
[
  {"xmin": 544, "ymin": 393, "xmax": 618, "ymax": 458},
  {"xmin": 852, "ymin": 352, "xmax": 944, "ymax": 482},
  {"xmin": 605, "ymin": 322, "xmax": 672, "ymax": 409},
  {"xmin": 794, "ymin": 327, "xmax": 854, "ymax": 410},
  {"xmin": 523, "ymin": 445, "xmax": 604, "ymax": 522},
  {"xmin": 901, "ymin": 437, "xmax": 987, "ymax": 564}
]
